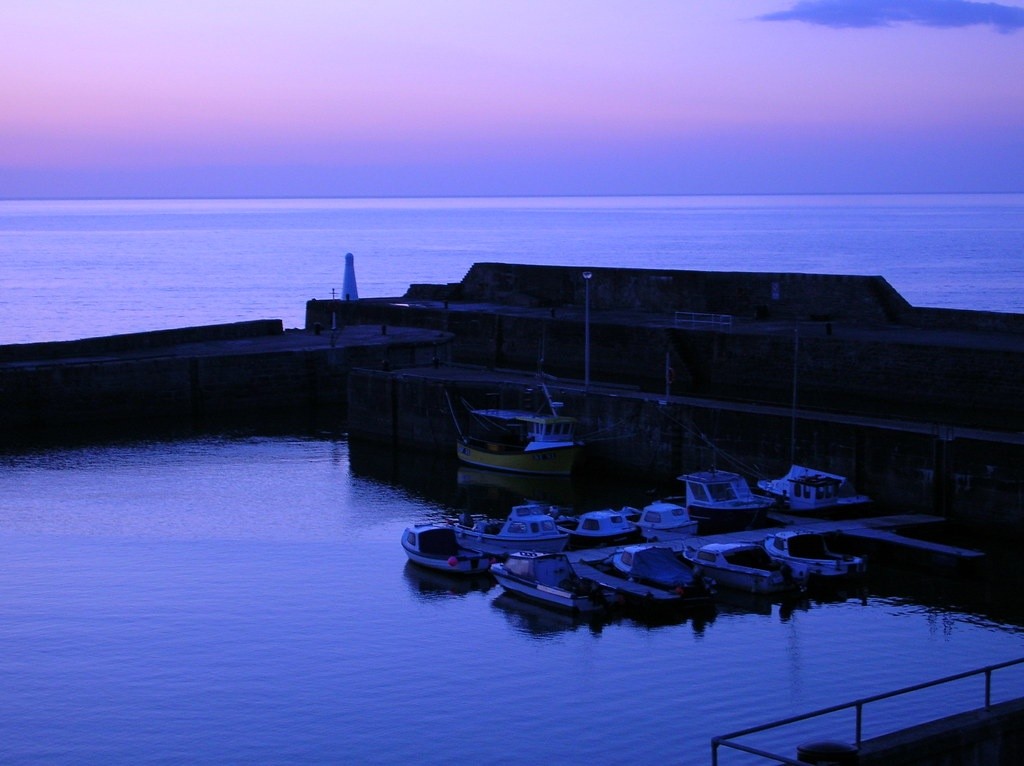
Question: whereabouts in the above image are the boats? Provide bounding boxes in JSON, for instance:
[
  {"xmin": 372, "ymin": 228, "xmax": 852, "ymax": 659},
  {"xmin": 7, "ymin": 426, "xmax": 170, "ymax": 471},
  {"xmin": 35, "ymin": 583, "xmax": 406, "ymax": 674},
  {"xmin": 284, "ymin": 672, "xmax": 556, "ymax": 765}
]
[
  {"xmin": 444, "ymin": 505, "xmax": 571, "ymax": 552},
  {"xmin": 760, "ymin": 530, "xmax": 867, "ymax": 587},
  {"xmin": 444, "ymin": 382, "xmax": 594, "ymax": 476},
  {"xmin": 400, "ymin": 523, "xmax": 489, "ymax": 575},
  {"xmin": 609, "ymin": 502, "xmax": 698, "ymax": 536},
  {"xmin": 651, "ymin": 465, "xmax": 770, "ymax": 536},
  {"xmin": 611, "ymin": 546, "xmax": 703, "ymax": 600},
  {"xmin": 770, "ymin": 474, "xmax": 875, "ymax": 520},
  {"xmin": 683, "ymin": 541, "xmax": 810, "ymax": 595},
  {"xmin": 554, "ymin": 510, "xmax": 643, "ymax": 550},
  {"xmin": 488, "ymin": 550, "xmax": 624, "ymax": 614}
]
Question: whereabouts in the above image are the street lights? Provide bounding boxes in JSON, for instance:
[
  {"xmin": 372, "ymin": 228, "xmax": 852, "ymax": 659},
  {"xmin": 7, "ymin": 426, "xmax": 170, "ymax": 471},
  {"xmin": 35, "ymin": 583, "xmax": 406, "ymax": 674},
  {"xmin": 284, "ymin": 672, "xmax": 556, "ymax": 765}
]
[{"xmin": 583, "ymin": 272, "xmax": 593, "ymax": 391}]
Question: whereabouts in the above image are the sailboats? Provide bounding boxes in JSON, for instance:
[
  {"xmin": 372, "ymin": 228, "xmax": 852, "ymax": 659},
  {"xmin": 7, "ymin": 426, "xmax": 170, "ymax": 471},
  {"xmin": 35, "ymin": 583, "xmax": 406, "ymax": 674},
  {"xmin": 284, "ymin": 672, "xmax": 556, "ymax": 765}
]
[{"xmin": 756, "ymin": 328, "xmax": 856, "ymax": 501}]
